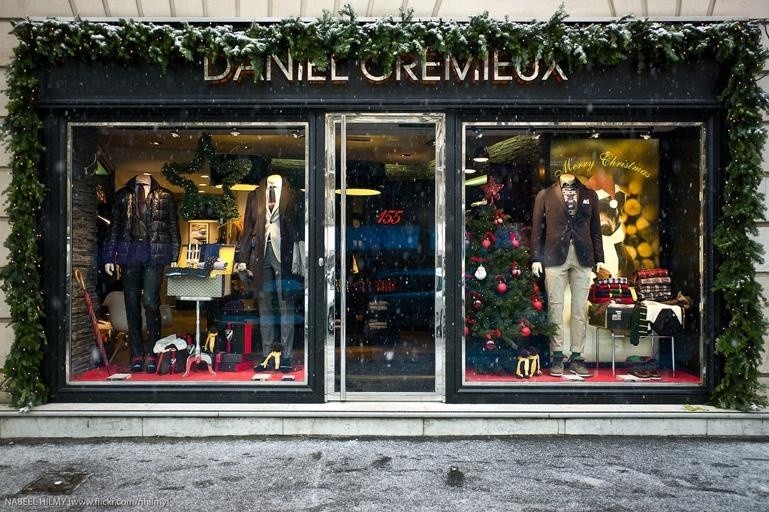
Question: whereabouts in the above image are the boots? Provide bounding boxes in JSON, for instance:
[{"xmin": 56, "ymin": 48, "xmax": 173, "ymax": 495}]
[
  {"xmin": 626, "ymin": 355, "xmax": 661, "ymax": 378},
  {"xmin": 567, "ymin": 355, "xmax": 591, "ymax": 378},
  {"xmin": 549, "ymin": 354, "xmax": 568, "ymax": 376}
]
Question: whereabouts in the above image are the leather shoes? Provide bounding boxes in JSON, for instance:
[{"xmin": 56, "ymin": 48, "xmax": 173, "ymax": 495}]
[
  {"xmin": 279, "ymin": 359, "xmax": 293, "ymax": 373},
  {"xmin": 255, "ymin": 362, "xmax": 272, "ymax": 373},
  {"xmin": 131, "ymin": 360, "xmax": 145, "ymax": 372},
  {"xmin": 146, "ymin": 361, "xmax": 157, "ymax": 374}
]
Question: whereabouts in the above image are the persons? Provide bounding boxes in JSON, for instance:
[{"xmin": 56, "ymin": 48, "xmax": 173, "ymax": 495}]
[
  {"xmin": 528, "ymin": 172, "xmax": 604, "ymax": 379},
  {"xmin": 101, "ymin": 174, "xmax": 183, "ymax": 375},
  {"xmin": 236, "ymin": 173, "xmax": 307, "ymax": 373}
]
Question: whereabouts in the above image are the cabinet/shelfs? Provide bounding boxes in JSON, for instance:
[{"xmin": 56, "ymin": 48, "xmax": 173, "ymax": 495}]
[{"xmin": 595, "ymin": 325, "xmax": 676, "ymax": 381}]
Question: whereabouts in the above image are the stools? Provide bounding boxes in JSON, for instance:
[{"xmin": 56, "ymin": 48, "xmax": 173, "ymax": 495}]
[{"xmin": 175, "ymin": 296, "xmax": 218, "ymax": 377}]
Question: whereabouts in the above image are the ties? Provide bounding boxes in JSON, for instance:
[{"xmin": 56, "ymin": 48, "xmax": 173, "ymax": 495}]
[
  {"xmin": 138, "ymin": 184, "xmax": 145, "ymax": 214},
  {"xmin": 269, "ymin": 187, "xmax": 277, "ymax": 212}
]
